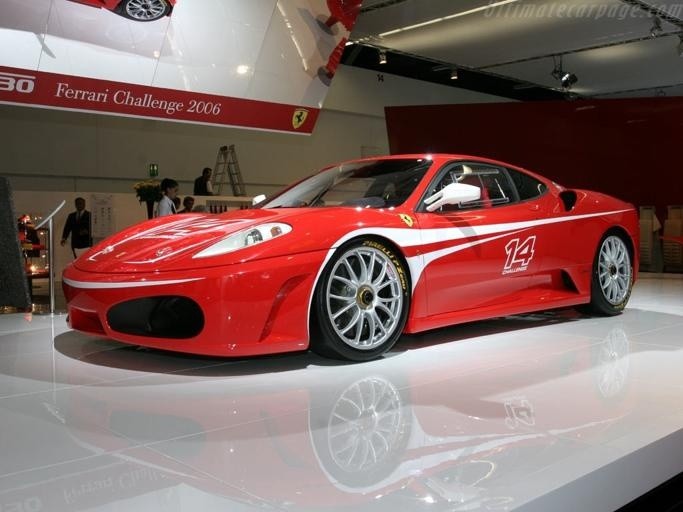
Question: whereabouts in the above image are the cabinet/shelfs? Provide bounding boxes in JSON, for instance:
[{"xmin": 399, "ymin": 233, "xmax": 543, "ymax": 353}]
[{"xmin": 16, "ymin": 215, "xmax": 56, "ymax": 313}]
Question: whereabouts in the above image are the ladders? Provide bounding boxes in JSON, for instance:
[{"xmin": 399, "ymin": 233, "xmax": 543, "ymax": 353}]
[{"xmin": 211, "ymin": 144, "xmax": 247, "ymax": 197}]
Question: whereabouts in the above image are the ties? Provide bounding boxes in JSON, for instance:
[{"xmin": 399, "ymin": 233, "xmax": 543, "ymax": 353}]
[
  {"xmin": 78, "ymin": 212, "xmax": 81, "ymax": 223},
  {"xmin": 171, "ymin": 204, "xmax": 176, "ymax": 213}
]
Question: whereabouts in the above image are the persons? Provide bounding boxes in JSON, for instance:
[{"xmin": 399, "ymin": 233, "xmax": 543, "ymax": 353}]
[
  {"xmin": 60, "ymin": 197, "xmax": 92, "ymax": 259},
  {"xmin": 177, "ymin": 196, "xmax": 194, "ymax": 214},
  {"xmin": 193, "ymin": 167, "xmax": 214, "ymax": 195},
  {"xmin": 172, "ymin": 197, "xmax": 179, "ymax": 209},
  {"xmin": 156, "ymin": 178, "xmax": 182, "ymax": 217}
]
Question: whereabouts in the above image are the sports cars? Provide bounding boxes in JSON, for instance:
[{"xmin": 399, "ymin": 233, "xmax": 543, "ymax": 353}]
[
  {"xmin": 66, "ymin": 0, "xmax": 178, "ymax": 25},
  {"xmin": 59, "ymin": 152, "xmax": 643, "ymax": 365},
  {"xmin": 37, "ymin": 313, "xmax": 645, "ymax": 512}
]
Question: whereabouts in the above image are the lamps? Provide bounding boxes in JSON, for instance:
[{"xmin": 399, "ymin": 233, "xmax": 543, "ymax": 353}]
[{"xmin": 551, "ymin": 54, "xmax": 578, "ymax": 88}]
